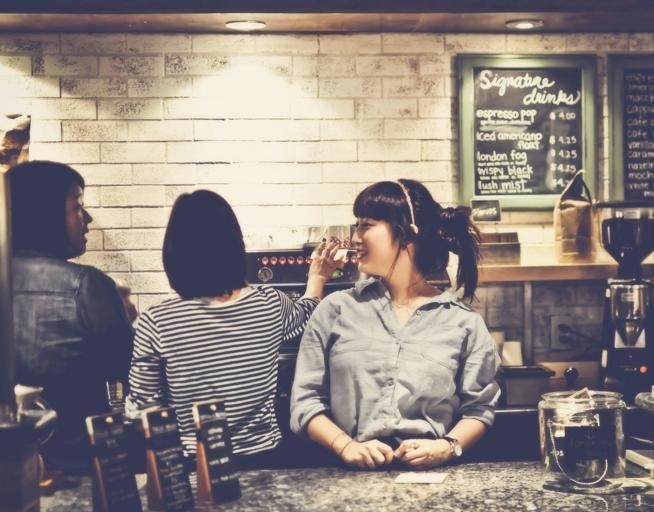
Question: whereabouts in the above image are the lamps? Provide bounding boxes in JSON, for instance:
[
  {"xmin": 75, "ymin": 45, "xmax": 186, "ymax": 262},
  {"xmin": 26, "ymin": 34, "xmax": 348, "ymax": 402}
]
[
  {"xmin": 224, "ymin": 19, "xmax": 267, "ymax": 33},
  {"xmin": 505, "ymin": 18, "xmax": 546, "ymax": 32}
]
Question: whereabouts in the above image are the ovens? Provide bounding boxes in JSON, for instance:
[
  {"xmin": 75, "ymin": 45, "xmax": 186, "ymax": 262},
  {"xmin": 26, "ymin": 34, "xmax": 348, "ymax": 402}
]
[{"xmin": 245, "ymin": 247, "xmax": 370, "ymax": 467}]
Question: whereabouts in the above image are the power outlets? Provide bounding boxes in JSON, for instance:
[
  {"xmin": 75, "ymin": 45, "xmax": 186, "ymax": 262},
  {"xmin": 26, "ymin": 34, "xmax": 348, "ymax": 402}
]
[{"xmin": 550, "ymin": 315, "xmax": 575, "ymax": 350}]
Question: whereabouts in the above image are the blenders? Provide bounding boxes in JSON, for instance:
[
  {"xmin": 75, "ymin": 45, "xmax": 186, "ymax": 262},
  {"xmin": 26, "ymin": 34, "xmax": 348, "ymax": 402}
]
[{"xmin": 597, "ymin": 198, "xmax": 653, "ymax": 406}]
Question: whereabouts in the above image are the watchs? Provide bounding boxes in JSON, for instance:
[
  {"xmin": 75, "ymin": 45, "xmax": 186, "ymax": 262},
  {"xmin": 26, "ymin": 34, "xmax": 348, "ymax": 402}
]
[{"xmin": 442, "ymin": 436, "xmax": 462, "ymax": 465}]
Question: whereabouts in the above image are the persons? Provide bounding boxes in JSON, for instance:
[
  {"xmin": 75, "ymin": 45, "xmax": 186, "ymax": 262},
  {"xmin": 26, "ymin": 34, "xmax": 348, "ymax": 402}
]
[
  {"xmin": 1, "ymin": 159, "xmax": 130, "ymax": 470},
  {"xmin": 125, "ymin": 187, "xmax": 341, "ymax": 466},
  {"xmin": 291, "ymin": 176, "xmax": 504, "ymax": 467}
]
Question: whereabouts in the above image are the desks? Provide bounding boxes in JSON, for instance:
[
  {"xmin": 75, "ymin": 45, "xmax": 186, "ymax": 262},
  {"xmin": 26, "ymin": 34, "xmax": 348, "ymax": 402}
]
[{"xmin": 40, "ymin": 459, "xmax": 654, "ymax": 512}]
